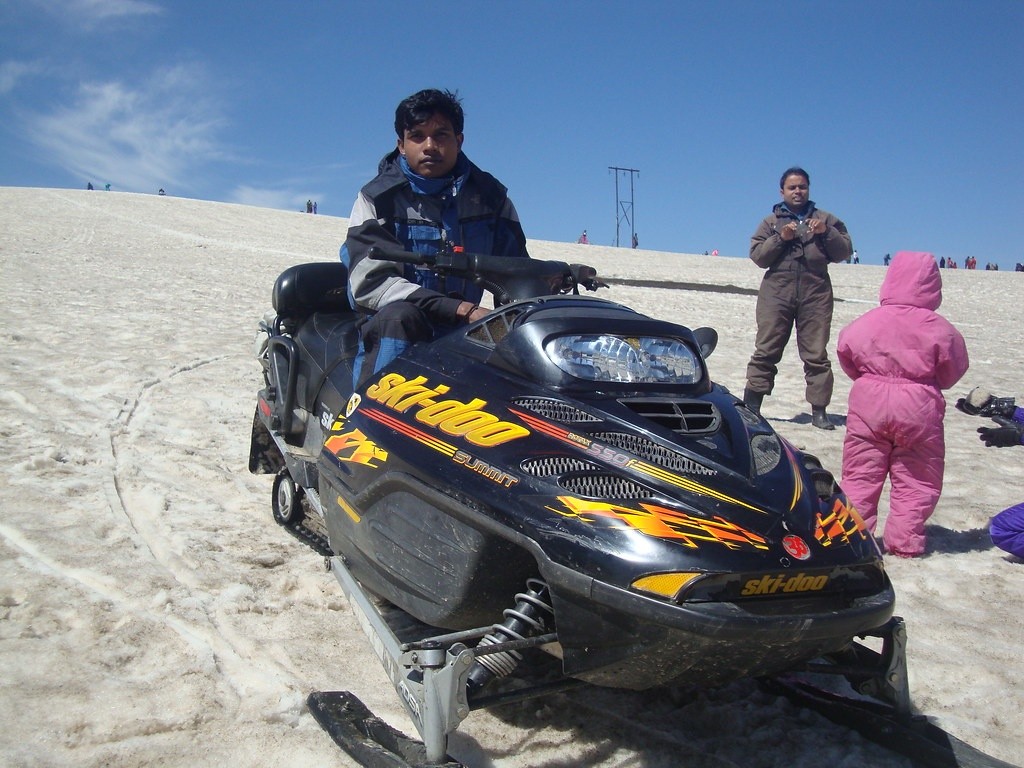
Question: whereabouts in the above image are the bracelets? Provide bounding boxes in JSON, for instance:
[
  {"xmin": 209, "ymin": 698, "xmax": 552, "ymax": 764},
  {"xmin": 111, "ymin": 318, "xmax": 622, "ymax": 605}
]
[{"xmin": 466, "ymin": 304, "xmax": 478, "ymax": 317}]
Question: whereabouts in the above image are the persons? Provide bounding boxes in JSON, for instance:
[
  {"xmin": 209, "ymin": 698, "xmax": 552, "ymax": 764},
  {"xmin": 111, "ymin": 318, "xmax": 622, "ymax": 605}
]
[
  {"xmin": 632, "ymin": 233, "xmax": 638, "ymax": 249},
  {"xmin": 106, "ymin": 183, "xmax": 111, "ymax": 191},
  {"xmin": 744, "ymin": 168, "xmax": 854, "ymax": 430},
  {"xmin": 307, "ymin": 200, "xmax": 317, "ymax": 214},
  {"xmin": 955, "ymin": 387, "xmax": 1024, "ymax": 560},
  {"xmin": 884, "ymin": 254, "xmax": 891, "ymax": 266},
  {"xmin": 705, "ymin": 251, "xmax": 708, "ymax": 255},
  {"xmin": 986, "ymin": 263, "xmax": 998, "ymax": 270},
  {"xmin": 965, "ymin": 256, "xmax": 976, "ymax": 269},
  {"xmin": 1016, "ymin": 263, "xmax": 1024, "ymax": 272},
  {"xmin": 836, "ymin": 252, "xmax": 969, "ymax": 558},
  {"xmin": 847, "ymin": 256, "xmax": 851, "ymax": 263},
  {"xmin": 340, "ymin": 89, "xmax": 531, "ymax": 395},
  {"xmin": 578, "ymin": 230, "xmax": 589, "ymax": 244},
  {"xmin": 712, "ymin": 249, "xmax": 719, "ymax": 256},
  {"xmin": 88, "ymin": 182, "xmax": 93, "ymax": 190},
  {"xmin": 854, "ymin": 250, "xmax": 859, "ymax": 264},
  {"xmin": 940, "ymin": 257, "xmax": 957, "ymax": 268}
]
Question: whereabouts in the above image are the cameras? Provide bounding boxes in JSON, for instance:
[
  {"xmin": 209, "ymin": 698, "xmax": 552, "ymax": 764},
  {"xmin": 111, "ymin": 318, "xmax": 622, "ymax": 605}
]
[{"xmin": 795, "ymin": 220, "xmax": 811, "ymax": 236}]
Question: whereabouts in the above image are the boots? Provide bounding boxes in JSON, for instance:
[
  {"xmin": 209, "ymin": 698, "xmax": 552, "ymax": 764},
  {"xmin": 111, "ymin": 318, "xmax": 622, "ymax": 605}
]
[
  {"xmin": 743, "ymin": 388, "xmax": 764, "ymax": 414},
  {"xmin": 812, "ymin": 404, "xmax": 834, "ymax": 430}
]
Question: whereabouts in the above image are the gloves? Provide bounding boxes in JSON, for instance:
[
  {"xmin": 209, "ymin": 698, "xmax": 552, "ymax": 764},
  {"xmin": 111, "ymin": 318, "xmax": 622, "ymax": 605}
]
[
  {"xmin": 955, "ymin": 386, "xmax": 1015, "ymax": 421},
  {"xmin": 976, "ymin": 415, "xmax": 1024, "ymax": 448}
]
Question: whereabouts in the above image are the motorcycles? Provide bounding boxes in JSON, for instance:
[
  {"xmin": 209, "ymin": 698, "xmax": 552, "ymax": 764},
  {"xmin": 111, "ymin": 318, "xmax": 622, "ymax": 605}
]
[{"xmin": 248, "ymin": 246, "xmax": 957, "ymax": 768}]
